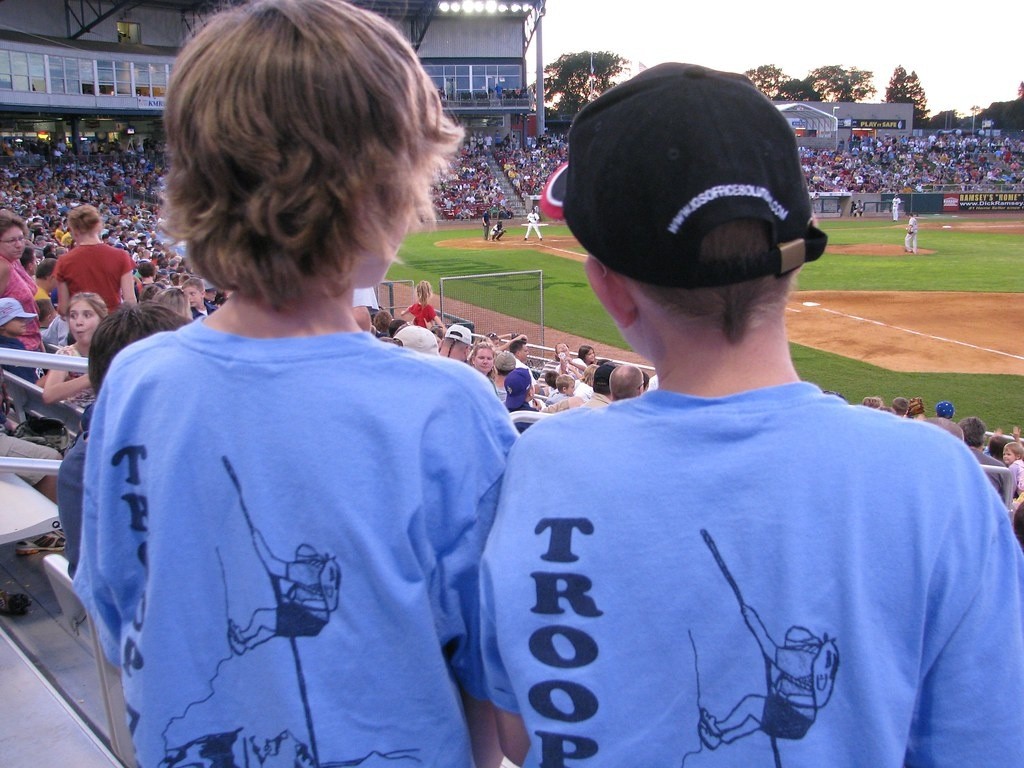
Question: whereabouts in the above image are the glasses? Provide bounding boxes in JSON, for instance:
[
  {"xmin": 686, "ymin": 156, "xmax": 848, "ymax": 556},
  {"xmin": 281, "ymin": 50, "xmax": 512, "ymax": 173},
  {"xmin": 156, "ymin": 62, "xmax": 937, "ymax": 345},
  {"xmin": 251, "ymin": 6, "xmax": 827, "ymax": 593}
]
[
  {"xmin": 490, "ymin": 333, "xmax": 496, "ymax": 337},
  {"xmin": 0, "ymin": 235, "xmax": 25, "ymax": 244}
]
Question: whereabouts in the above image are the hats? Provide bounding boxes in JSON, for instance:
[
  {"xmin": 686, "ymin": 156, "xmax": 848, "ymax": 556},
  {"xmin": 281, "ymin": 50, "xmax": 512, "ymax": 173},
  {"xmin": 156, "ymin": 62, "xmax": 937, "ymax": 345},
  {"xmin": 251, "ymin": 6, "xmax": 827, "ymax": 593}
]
[
  {"xmin": 445, "ymin": 324, "xmax": 471, "ymax": 345},
  {"xmin": 393, "ymin": 326, "xmax": 440, "ymax": 357},
  {"xmin": 936, "ymin": 401, "xmax": 955, "ymax": 418},
  {"xmin": 504, "ymin": 368, "xmax": 531, "ymax": 408},
  {"xmin": 593, "ymin": 365, "xmax": 616, "ymax": 386},
  {"xmin": 0, "ymin": 298, "xmax": 38, "ymax": 327},
  {"xmin": 539, "ymin": 61, "xmax": 829, "ymax": 290}
]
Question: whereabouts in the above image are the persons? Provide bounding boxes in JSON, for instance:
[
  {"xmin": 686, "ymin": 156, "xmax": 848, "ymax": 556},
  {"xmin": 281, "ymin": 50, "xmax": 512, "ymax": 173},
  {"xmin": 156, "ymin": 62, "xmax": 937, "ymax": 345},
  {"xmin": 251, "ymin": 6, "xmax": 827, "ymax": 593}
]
[
  {"xmin": 432, "ymin": 144, "xmax": 571, "ymax": 222},
  {"xmin": 490, "ymin": 220, "xmax": 506, "ymax": 241},
  {"xmin": 527, "ymin": 134, "xmax": 551, "ymax": 149},
  {"xmin": 69, "ymin": 0, "xmax": 520, "ymax": 768},
  {"xmin": 863, "ymin": 396, "xmax": 1024, "ymax": 546},
  {"xmin": 503, "ymin": 368, "xmax": 541, "ymax": 433},
  {"xmin": 850, "ymin": 200, "xmax": 863, "ymax": 217},
  {"xmin": 525, "ymin": 209, "xmax": 542, "ymax": 240},
  {"xmin": 905, "ymin": 214, "xmax": 917, "ymax": 253},
  {"xmin": 494, "ymin": 84, "xmax": 503, "ymax": 106},
  {"xmin": 560, "ymin": 139, "xmax": 565, "ymax": 147},
  {"xmin": 798, "ymin": 132, "xmax": 1024, "ymax": 192},
  {"xmin": 515, "ymin": 87, "xmax": 520, "ymax": 96},
  {"xmin": 353, "ymin": 305, "xmax": 650, "ymax": 415},
  {"xmin": 479, "ymin": 62, "xmax": 1024, "ymax": 768},
  {"xmin": 0, "ymin": 138, "xmax": 237, "ymax": 617},
  {"xmin": 469, "ymin": 130, "xmax": 518, "ymax": 151},
  {"xmin": 402, "ymin": 280, "xmax": 445, "ymax": 333},
  {"xmin": 481, "ymin": 208, "xmax": 490, "ymax": 240},
  {"xmin": 813, "ymin": 193, "xmax": 819, "ymax": 213},
  {"xmin": 892, "ymin": 195, "xmax": 901, "ymax": 222}
]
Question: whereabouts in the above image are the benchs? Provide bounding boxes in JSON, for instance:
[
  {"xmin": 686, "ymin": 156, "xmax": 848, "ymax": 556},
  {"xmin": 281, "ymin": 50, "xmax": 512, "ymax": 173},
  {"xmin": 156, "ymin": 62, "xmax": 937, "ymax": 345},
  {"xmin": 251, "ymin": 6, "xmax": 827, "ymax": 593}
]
[
  {"xmin": 857, "ymin": 133, "xmax": 1024, "ymax": 196},
  {"xmin": 0, "ymin": 313, "xmax": 611, "ymax": 768},
  {"xmin": 427, "ymin": 139, "xmax": 538, "ymax": 218}
]
